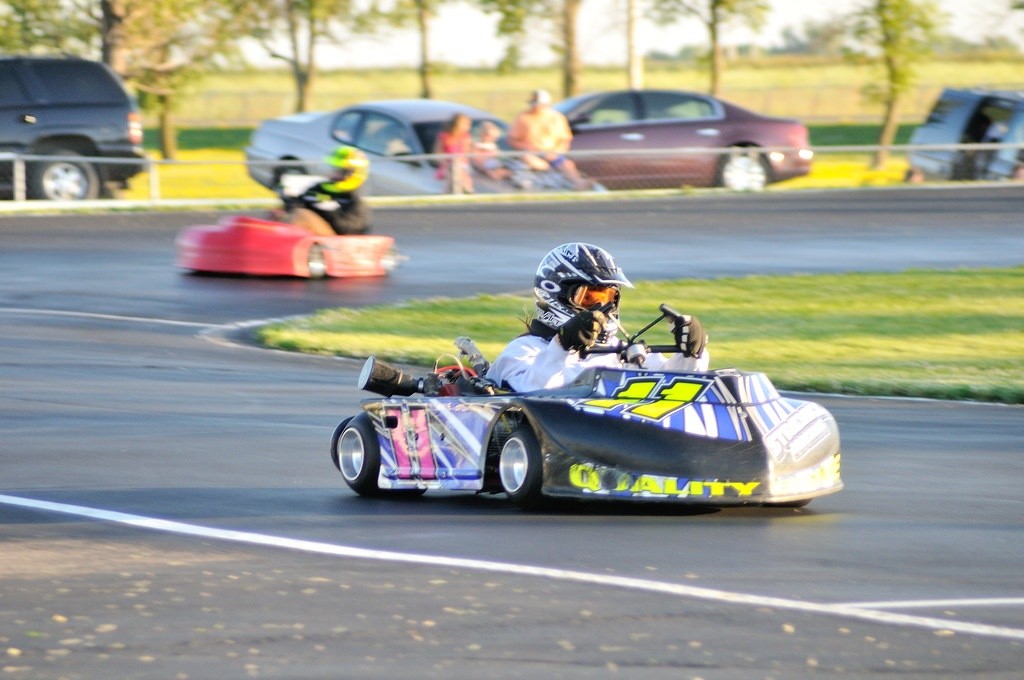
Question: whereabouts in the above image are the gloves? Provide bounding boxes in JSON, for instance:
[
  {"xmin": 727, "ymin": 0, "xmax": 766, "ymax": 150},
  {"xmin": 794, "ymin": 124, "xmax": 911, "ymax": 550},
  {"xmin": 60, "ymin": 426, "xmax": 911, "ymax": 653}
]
[
  {"xmin": 557, "ymin": 301, "xmax": 609, "ymax": 352},
  {"xmin": 671, "ymin": 314, "xmax": 709, "ymax": 359}
]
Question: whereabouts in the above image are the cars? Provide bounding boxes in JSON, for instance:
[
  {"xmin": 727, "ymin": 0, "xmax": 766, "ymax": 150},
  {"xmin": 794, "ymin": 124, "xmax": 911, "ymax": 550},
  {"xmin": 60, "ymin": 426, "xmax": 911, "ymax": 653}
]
[
  {"xmin": 554, "ymin": 85, "xmax": 816, "ymax": 192},
  {"xmin": 242, "ymin": 97, "xmax": 611, "ymax": 196},
  {"xmin": 903, "ymin": 83, "xmax": 1024, "ymax": 184}
]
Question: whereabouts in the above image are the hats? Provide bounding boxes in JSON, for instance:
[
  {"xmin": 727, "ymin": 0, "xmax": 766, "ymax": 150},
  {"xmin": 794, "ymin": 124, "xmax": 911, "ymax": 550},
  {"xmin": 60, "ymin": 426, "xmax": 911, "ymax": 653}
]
[{"xmin": 524, "ymin": 90, "xmax": 551, "ymax": 106}]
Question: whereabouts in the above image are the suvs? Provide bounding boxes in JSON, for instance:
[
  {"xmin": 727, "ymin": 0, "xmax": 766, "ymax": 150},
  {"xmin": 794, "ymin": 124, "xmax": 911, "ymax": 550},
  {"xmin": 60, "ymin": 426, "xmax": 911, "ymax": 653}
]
[{"xmin": 0, "ymin": 55, "xmax": 152, "ymax": 203}]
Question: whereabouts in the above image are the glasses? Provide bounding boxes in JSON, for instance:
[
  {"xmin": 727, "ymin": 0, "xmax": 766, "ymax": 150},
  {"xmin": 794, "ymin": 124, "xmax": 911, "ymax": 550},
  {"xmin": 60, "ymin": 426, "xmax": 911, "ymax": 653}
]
[{"xmin": 559, "ymin": 282, "xmax": 620, "ymax": 313}]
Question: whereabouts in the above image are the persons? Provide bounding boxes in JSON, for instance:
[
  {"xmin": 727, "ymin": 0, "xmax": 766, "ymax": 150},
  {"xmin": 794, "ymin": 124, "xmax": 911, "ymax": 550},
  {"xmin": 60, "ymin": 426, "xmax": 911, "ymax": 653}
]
[
  {"xmin": 433, "ymin": 115, "xmax": 473, "ymax": 194},
  {"xmin": 902, "ymin": 168, "xmax": 924, "ymax": 182},
  {"xmin": 473, "ymin": 122, "xmax": 510, "ymax": 181},
  {"xmin": 286, "ymin": 146, "xmax": 369, "ymax": 235},
  {"xmin": 486, "ymin": 243, "xmax": 709, "ymax": 392},
  {"xmin": 508, "ymin": 90, "xmax": 596, "ymax": 190}
]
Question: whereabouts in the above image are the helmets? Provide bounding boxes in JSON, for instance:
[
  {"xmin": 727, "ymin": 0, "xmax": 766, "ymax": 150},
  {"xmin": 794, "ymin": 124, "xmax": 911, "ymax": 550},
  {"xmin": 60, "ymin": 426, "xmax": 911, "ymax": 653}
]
[
  {"xmin": 320, "ymin": 146, "xmax": 371, "ymax": 194},
  {"xmin": 527, "ymin": 244, "xmax": 635, "ymax": 349}
]
